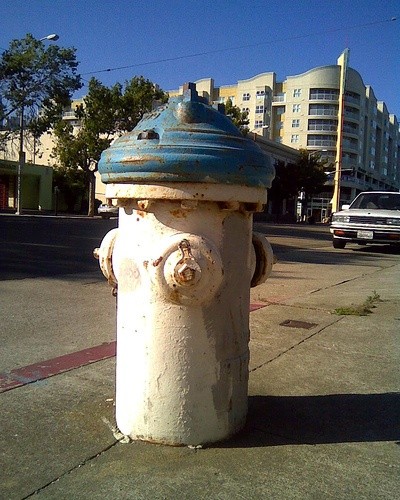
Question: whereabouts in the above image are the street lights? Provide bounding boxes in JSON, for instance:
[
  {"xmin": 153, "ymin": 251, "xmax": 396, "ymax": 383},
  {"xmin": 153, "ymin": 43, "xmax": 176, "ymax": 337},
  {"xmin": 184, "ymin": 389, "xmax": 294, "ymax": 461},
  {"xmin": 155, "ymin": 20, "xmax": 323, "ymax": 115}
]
[{"xmin": 15, "ymin": 32, "xmax": 60, "ymax": 214}]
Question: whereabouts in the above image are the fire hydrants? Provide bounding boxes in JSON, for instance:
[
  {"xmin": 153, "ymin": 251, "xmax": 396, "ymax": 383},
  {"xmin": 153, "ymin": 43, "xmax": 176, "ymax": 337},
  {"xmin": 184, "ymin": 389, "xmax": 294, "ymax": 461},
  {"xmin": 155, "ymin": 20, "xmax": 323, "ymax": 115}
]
[{"xmin": 94, "ymin": 82, "xmax": 277, "ymax": 447}]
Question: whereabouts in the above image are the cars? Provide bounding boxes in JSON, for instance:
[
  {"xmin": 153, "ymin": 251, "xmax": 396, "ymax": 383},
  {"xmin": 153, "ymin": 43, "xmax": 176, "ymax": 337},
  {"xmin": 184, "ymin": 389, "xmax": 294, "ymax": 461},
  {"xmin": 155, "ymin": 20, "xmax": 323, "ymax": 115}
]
[
  {"xmin": 330, "ymin": 192, "xmax": 400, "ymax": 251},
  {"xmin": 98, "ymin": 204, "xmax": 119, "ymax": 220}
]
